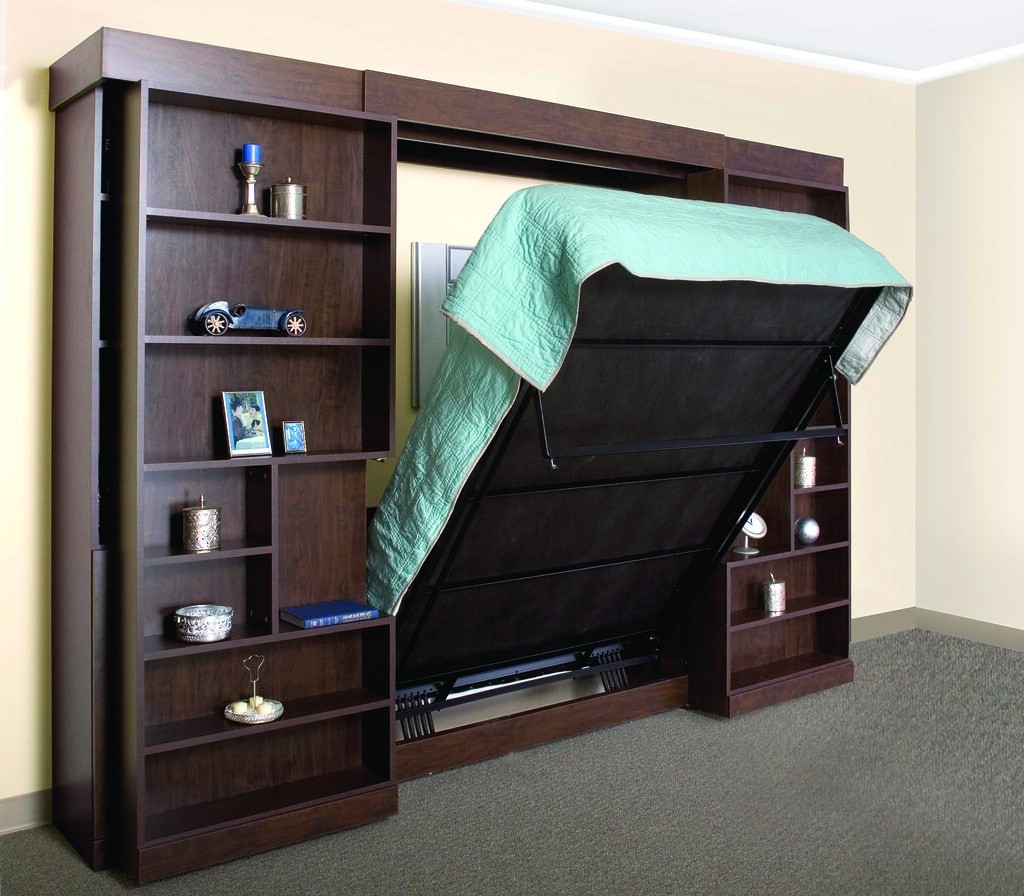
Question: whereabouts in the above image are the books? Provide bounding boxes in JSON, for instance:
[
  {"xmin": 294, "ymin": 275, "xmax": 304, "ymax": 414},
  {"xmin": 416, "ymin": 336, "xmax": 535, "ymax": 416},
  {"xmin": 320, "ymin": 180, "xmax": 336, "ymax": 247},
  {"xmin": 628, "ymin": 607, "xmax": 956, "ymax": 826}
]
[{"xmin": 279, "ymin": 597, "xmax": 379, "ymax": 629}]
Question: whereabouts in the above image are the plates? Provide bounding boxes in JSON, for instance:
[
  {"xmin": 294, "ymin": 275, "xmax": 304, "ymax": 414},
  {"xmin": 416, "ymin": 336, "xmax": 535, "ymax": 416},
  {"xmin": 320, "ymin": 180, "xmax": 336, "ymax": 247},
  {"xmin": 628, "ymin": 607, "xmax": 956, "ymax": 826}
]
[{"xmin": 224, "ymin": 698, "xmax": 284, "ymax": 724}]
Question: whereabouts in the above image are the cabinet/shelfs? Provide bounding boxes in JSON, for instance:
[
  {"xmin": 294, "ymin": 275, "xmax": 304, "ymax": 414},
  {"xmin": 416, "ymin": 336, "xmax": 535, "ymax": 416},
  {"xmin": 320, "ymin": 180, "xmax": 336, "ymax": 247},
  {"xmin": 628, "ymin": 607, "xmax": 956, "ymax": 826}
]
[{"xmin": 49, "ymin": 27, "xmax": 914, "ymax": 889}]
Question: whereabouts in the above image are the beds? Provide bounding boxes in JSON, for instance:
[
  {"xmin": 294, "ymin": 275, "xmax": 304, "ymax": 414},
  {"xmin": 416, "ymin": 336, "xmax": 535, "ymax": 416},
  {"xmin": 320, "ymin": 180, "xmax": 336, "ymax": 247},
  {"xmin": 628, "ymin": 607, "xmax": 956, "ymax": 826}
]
[{"xmin": 365, "ymin": 182, "xmax": 912, "ymax": 744}]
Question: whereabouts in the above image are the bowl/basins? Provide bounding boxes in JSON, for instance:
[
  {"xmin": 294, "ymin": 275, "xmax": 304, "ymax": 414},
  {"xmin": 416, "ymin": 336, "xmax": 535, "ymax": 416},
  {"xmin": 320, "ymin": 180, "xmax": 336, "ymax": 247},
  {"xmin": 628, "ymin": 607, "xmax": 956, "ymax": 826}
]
[{"xmin": 175, "ymin": 605, "xmax": 233, "ymax": 642}]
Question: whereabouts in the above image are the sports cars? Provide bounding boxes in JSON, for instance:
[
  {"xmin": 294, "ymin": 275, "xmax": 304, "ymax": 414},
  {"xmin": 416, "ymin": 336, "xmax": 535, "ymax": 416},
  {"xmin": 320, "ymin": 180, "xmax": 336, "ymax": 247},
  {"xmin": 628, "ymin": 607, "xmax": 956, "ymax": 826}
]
[{"xmin": 186, "ymin": 300, "xmax": 307, "ymax": 337}]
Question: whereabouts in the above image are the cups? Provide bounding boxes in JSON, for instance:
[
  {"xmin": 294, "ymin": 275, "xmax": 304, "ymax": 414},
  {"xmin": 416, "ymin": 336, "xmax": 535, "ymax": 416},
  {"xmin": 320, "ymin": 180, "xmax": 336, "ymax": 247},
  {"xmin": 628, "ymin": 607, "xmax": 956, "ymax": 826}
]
[
  {"xmin": 182, "ymin": 492, "xmax": 222, "ymax": 551},
  {"xmin": 269, "ymin": 176, "xmax": 308, "ymax": 219},
  {"xmin": 762, "ymin": 572, "xmax": 786, "ymax": 612},
  {"xmin": 794, "ymin": 447, "xmax": 816, "ymax": 489}
]
